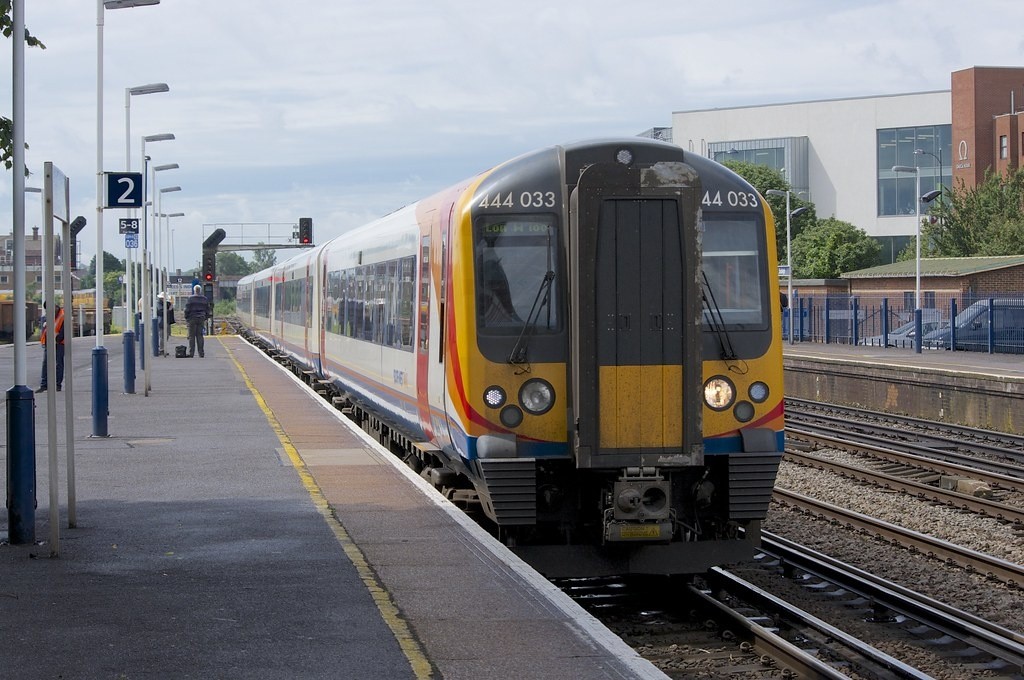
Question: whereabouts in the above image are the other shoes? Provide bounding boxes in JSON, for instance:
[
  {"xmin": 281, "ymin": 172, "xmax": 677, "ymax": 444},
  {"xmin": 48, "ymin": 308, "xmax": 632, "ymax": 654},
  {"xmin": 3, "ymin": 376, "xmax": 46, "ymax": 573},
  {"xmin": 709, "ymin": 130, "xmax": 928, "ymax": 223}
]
[
  {"xmin": 35, "ymin": 386, "xmax": 47, "ymax": 393},
  {"xmin": 160, "ymin": 350, "xmax": 169, "ymax": 355},
  {"xmin": 56, "ymin": 383, "xmax": 62, "ymax": 390},
  {"xmin": 199, "ymin": 354, "xmax": 204, "ymax": 357},
  {"xmin": 186, "ymin": 355, "xmax": 193, "ymax": 358}
]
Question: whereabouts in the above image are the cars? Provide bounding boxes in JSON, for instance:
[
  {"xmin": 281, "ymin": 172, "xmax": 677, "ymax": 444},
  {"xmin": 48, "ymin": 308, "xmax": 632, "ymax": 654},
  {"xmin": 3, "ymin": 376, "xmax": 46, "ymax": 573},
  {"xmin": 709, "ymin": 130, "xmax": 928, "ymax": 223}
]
[{"xmin": 857, "ymin": 319, "xmax": 951, "ymax": 348}]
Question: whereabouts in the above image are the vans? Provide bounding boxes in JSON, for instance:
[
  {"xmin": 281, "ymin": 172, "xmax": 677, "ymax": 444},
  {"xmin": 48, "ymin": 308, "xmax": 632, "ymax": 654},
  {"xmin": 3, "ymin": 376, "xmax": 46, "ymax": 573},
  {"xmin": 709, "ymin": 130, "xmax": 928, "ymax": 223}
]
[{"xmin": 922, "ymin": 300, "xmax": 1024, "ymax": 353}]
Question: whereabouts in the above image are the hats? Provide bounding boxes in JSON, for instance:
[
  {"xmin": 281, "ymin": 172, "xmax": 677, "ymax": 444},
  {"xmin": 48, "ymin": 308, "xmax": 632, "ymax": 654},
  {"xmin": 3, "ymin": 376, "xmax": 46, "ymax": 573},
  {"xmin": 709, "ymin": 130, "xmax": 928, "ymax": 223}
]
[{"xmin": 157, "ymin": 292, "xmax": 171, "ymax": 299}]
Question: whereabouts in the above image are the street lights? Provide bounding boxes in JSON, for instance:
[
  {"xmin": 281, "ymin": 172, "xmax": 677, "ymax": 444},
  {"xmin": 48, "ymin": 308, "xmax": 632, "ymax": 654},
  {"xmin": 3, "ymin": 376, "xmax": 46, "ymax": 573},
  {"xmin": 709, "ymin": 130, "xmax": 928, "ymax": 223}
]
[
  {"xmin": 91, "ymin": 0, "xmax": 162, "ymax": 435},
  {"xmin": 158, "ymin": 187, "xmax": 181, "ymax": 294},
  {"xmin": 24, "ymin": 187, "xmax": 47, "ymax": 334},
  {"xmin": 893, "ymin": 166, "xmax": 942, "ymax": 357},
  {"xmin": 166, "ymin": 213, "xmax": 185, "ymax": 282},
  {"xmin": 124, "ymin": 83, "xmax": 169, "ymax": 394},
  {"xmin": 913, "ymin": 148, "xmax": 942, "ymax": 238},
  {"xmin": 765, "ymin": 189, "xmax": 807, "ymax": 346},
  {"xmin": 140, "ymin": 133, "xmax": 175, "ymax": 372},
  {"xmin": 151, "ymin": 164, "xmax": 179, "ymax": 357}
]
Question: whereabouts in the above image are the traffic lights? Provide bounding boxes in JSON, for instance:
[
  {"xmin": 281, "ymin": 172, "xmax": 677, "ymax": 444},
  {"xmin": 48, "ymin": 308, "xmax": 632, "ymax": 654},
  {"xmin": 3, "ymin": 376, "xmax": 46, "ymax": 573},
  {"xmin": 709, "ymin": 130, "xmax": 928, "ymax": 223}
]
[
  {"xmin": 203, "ymin": 229, "xmax": 226, "ymax": 251},
  {"xmin": 300, "ymin": 218, "xmax": 312, "ymax": 244},
  {"xmin": 70, "ymin": 216, "xmax": 86, "ymax": 238},
  {"xmin": 70, "ymin": 240, "xmax": 76, "ymax": 267},
  {"xmin": 203, "ymin": 253, "xmax": 216, "ymax": 282}
]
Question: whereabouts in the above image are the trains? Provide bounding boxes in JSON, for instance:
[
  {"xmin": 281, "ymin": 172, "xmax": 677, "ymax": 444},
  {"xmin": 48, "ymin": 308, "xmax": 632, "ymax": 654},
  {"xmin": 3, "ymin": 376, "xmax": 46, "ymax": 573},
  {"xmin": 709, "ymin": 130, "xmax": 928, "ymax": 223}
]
[{"xmin": 237, "ymin": 136, "xmax": 786, "ymax": 581}]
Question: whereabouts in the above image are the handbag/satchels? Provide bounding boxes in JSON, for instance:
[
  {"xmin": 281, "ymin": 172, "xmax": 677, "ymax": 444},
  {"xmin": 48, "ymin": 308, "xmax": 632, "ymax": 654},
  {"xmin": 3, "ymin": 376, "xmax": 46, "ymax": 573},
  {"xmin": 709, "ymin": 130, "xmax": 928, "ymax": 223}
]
[{"xmin": 176, "ymin": 345, "xmax": 187, "ymax": 358}]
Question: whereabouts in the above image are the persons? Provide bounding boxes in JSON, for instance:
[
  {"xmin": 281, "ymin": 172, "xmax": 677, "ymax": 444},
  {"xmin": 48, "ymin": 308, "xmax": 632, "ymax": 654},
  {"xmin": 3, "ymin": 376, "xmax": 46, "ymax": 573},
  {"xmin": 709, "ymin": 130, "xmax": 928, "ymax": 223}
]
[
  {"xmin": 155, "ymin": 291, "xmax": 174, "ymax": 356},
  {"xmin": 183, "ymin": 285, "xmax": 211, "ymax": 358},
  {"xmin": 35, "ymin": 301, "xmax": 65, "ymax": 394}
]
